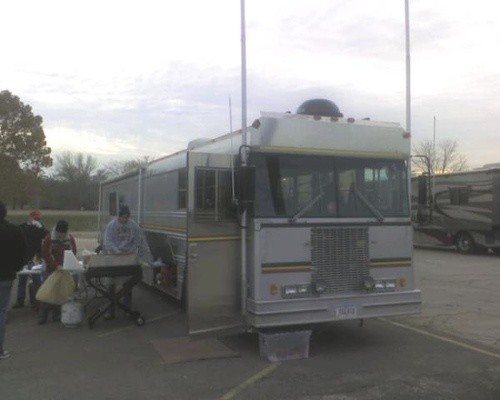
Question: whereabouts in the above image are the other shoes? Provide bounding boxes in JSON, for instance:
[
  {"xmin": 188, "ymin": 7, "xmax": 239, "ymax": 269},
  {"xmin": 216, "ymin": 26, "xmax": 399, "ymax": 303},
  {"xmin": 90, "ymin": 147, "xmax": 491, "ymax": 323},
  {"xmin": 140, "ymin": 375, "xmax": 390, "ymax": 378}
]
[
  {"xmin": 104, "ymin": 312, "xmax": 115, "ymax": 320},
  {"xmin": 38, "ymin": 318, "xmax": 45, "ymax": 324},
  {"xmin": 13, "ymin": 301, "xmax": 24, "ymax": 308},
  {"xmin": 125, "ymin": 306, "xmax": 131, "ymax": 317}
]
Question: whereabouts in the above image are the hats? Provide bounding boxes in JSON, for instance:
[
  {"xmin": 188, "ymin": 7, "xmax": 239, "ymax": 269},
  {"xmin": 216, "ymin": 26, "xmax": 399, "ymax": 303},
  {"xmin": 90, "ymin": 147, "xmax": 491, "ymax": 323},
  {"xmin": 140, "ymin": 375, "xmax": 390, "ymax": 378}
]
[
  {"xmin": 118, "ymin": 205, "xmax": 129, "ymax": 214},
  {"xmin": 56, "ymin": 220, "xmax": 69, "ymax": 233},
  {"xmin": 30, "ymin": 210, "xmax": 40, "ymax": 219}
]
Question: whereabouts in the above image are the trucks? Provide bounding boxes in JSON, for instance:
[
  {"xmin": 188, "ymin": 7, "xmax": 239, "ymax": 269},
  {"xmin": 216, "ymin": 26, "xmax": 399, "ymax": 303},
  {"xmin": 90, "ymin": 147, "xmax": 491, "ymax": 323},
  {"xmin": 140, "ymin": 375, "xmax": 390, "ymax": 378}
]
[
  {"xmin": 98, "ymin": 99, "xmax": 431, "ymax": 332},
  {"xmin": 412, "ymin": 168, "xmax": 500, "ymax": 255}
]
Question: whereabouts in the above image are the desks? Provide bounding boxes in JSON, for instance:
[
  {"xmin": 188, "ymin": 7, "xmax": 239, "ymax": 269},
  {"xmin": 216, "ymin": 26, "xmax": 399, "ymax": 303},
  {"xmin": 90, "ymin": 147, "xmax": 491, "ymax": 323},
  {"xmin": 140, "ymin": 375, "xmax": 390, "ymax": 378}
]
[{"xmin": 83, "ymin": 265, "xmax": 144, "ymax": 328}]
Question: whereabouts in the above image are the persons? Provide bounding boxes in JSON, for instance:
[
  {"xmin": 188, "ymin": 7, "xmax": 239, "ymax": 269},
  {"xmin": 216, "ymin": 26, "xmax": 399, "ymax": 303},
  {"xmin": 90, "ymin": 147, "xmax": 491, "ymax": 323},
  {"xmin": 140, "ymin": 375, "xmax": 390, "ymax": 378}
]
[
  {"xmin": 0, "ymin": 202, "xmax": 16, "ymax": 358},
  {"xmin": 40, "ymin": 220, "xmax": 77, "ymax": 323},
  {"xmin": 12, "ymin": 209, "xmax": 49, "ymax": 307},
  {"xmin": 105, "ymin": 206, "xmax": 151, "ymax": 296}
]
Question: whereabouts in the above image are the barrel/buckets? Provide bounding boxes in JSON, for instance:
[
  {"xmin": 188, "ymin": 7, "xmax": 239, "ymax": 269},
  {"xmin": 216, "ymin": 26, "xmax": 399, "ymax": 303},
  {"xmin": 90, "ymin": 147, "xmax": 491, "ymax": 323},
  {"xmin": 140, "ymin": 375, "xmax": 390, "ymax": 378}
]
[{"xmin": 61, "ymin": 302, "xmax": 84, "ymax": 324}]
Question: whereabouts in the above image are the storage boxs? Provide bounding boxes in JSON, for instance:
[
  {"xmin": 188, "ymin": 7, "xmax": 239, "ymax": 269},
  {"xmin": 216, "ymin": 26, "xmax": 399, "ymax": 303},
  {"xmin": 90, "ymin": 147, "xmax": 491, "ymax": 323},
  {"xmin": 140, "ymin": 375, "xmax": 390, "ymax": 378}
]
[{"xmin": 259, "ymin": 329, "xmax": 313, "ymax": 362}]
